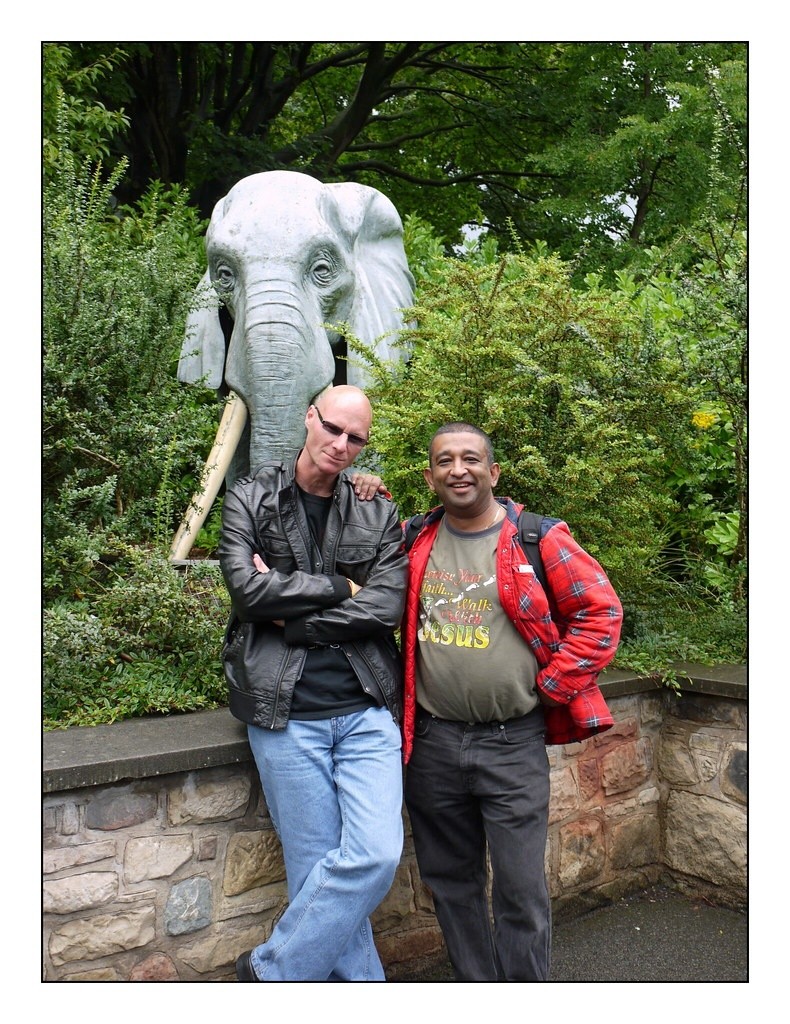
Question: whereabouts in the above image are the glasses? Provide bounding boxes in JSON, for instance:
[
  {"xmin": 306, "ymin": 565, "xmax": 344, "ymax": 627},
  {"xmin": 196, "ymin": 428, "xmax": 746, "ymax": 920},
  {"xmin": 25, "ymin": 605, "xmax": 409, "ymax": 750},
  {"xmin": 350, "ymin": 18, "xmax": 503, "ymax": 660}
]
[{"xmin": 315, "ymin": 407, "xmax": 370, "ymax": 447}]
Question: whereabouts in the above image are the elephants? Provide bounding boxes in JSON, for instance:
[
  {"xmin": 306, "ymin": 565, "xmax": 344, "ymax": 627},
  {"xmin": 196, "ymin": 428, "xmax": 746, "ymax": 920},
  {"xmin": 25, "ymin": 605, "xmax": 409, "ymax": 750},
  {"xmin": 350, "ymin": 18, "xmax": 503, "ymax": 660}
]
[{"xmin": 165, "ymin": 170, "xmax": 418, "ymax": 563}]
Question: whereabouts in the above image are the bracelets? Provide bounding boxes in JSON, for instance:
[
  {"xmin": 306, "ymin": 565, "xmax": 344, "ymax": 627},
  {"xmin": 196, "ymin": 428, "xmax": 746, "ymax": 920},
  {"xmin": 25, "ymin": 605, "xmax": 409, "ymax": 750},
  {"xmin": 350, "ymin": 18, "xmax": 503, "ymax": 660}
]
[{"xmin": 346, "ymin": 576, "xmax": 356, "ymax": 602}]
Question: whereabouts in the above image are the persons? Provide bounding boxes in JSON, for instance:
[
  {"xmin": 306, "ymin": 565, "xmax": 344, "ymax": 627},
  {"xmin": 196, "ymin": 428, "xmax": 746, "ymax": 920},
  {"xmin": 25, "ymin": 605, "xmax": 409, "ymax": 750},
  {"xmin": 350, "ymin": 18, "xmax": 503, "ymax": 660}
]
[
  {"xmin": 350, "ymin": 421, "xmax": 623, "ymax": 981},
  {"xmin": 219, "ymin": 385, "xmax": 410, "ymax": 981}
]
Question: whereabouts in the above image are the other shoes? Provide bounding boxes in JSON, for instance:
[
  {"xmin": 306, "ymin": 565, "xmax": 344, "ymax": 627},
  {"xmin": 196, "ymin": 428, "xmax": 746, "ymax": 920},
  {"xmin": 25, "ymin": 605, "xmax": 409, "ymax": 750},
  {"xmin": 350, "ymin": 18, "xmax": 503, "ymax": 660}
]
[{"xmin": 235, "ymin": 950, "xmax": 260, "ymax": 981}]
